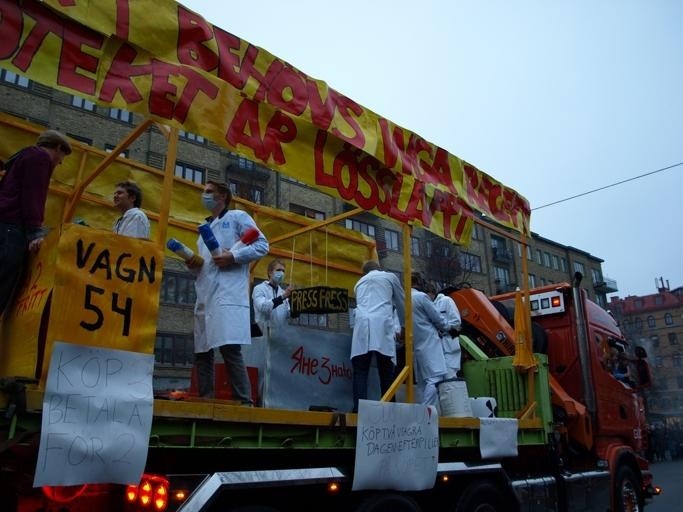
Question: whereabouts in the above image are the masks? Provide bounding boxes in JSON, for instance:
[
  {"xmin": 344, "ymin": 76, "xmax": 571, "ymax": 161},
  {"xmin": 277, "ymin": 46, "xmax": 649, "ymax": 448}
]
[
  {"xmin": 202, "ymin": 193, "xmax": 218, "ymax": 210},
  {"xmin": 272, "ymin": 271, "xmax": 284, "ymax": 283}
]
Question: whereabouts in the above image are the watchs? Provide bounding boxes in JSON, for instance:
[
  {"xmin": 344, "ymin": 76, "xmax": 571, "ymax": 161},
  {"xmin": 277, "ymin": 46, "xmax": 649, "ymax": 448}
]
[{"xmin": 280, "ymin": 294, "xmax": 284, "ymax": 300}]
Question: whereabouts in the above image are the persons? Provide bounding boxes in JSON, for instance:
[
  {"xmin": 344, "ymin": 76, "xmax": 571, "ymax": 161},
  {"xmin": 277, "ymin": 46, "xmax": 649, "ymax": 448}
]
[
  {"xmin": 251, "ymin": 258, "xmax": 295, "ymax": 333},
  {"xmin": 113, "ymin": 179, "xmax": 149, "ymax": 239},
  {"xmin": 0, "ymin": 131, "xmax": 72, "ymax": 313},
  {"xmin": 410, "ymin": 270, "xmax": 448, "ymax": 407},
  {"xmin": 348, "ymin": 261, "xmax": 404, "ymax": 413},
  {"xmin": 646, "ymin": 420, "xmax": 683, "ymax": 465},
  {"xmin": 423, "ymin": 282, "xmax": 461, "ymax": 380},
  {"xmin": 607, "ymin": 347, "xmax": 631, "ymax": 380},
  {"xmin": 184, "ymin": 180, "xmax": 270, "ymax": 404}
]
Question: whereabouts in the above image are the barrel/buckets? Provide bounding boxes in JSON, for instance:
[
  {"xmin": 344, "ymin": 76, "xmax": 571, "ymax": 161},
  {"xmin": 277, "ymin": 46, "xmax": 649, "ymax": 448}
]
[{"xmin": 438, "ymin": 380, "xmax": 472, "ymax": 417}]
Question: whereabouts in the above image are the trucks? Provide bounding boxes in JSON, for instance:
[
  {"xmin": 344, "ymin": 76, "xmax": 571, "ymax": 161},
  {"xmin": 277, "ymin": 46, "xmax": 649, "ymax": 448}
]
[{"xmin": 0, "ymin": 111, "xmax": 661, "ymax": 511}]
